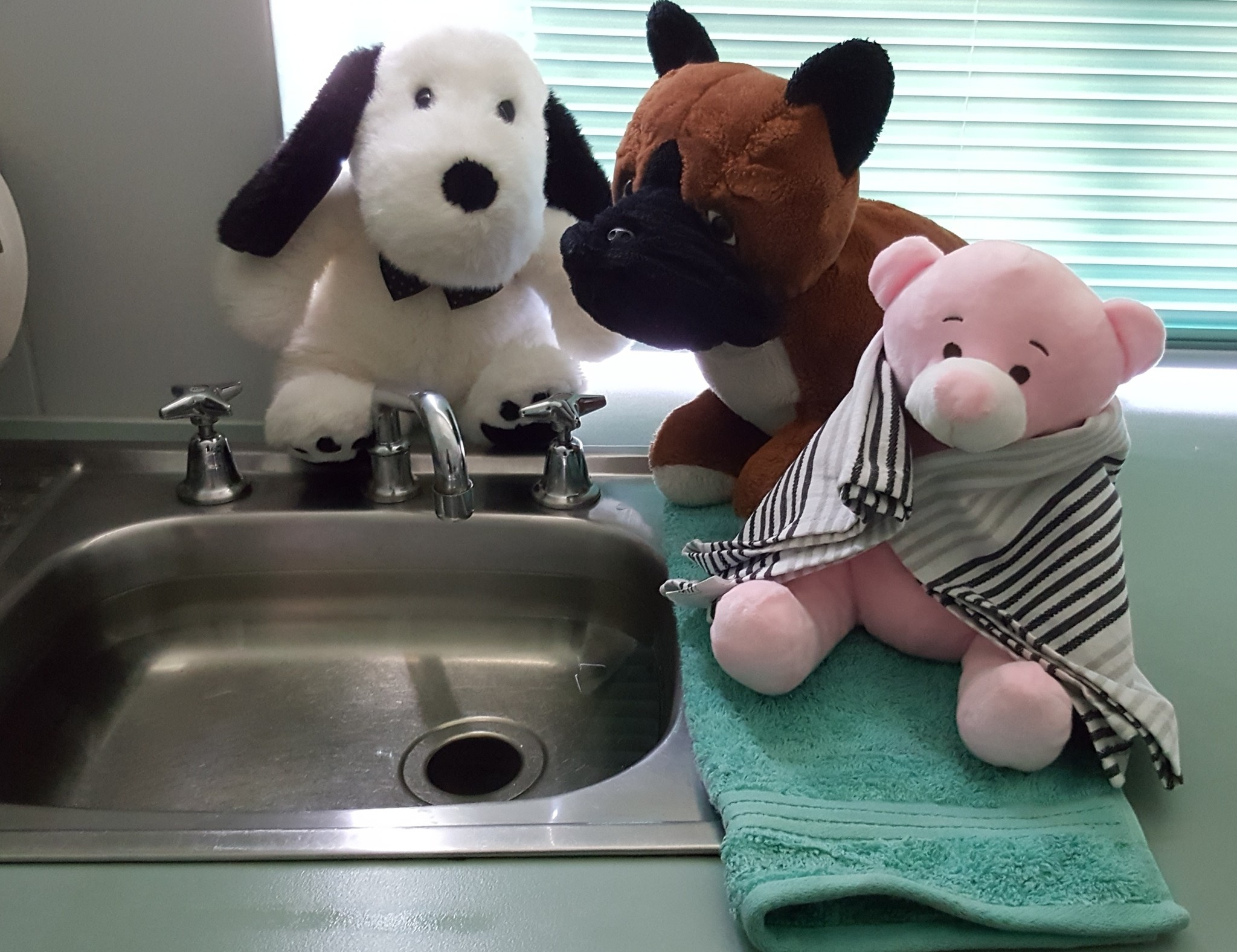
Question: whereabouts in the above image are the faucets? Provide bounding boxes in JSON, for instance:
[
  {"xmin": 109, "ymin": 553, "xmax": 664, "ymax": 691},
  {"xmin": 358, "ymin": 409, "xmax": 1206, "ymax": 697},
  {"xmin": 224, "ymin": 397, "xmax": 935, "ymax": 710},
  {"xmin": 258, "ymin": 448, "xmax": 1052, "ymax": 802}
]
[{"xmin": 353, "ymin": 389, "xmax": 477, "ymax": 522}]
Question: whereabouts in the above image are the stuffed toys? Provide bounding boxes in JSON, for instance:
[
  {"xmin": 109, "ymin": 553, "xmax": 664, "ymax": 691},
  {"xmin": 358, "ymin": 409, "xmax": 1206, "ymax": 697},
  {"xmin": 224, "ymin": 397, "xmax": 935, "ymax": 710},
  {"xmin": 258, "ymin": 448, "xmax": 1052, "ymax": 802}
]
[
  {"xmin": 709, "ymin": 235, "xmax": 1166, "ymax": 773},
  {"xmin": 560, "ymin": 0, "xmax": 971, "ymax": 527},
  {"xmin": 217, "ymin": 25, "xmax": 632, "ymax": 462}
]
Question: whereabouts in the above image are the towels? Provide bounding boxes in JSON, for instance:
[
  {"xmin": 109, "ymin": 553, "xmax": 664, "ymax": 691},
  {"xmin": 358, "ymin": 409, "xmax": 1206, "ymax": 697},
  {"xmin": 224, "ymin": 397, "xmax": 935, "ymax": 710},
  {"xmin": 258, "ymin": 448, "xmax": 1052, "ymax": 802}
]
[
  {"xmin": 659, "ymin": 325, "xmax": 1183, "ymax": 790},
  {"xmin": 603, "ymin": 500, "xmax": 1190, "ymax": 952}
]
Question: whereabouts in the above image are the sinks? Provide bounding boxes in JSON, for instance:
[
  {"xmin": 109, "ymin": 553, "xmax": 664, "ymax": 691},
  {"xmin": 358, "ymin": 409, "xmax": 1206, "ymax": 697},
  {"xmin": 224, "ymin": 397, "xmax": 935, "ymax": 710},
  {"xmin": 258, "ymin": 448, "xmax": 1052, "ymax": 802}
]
[{"xmin": 0, "ymin": 439, "xmax": 725, "ymax": 861}]
